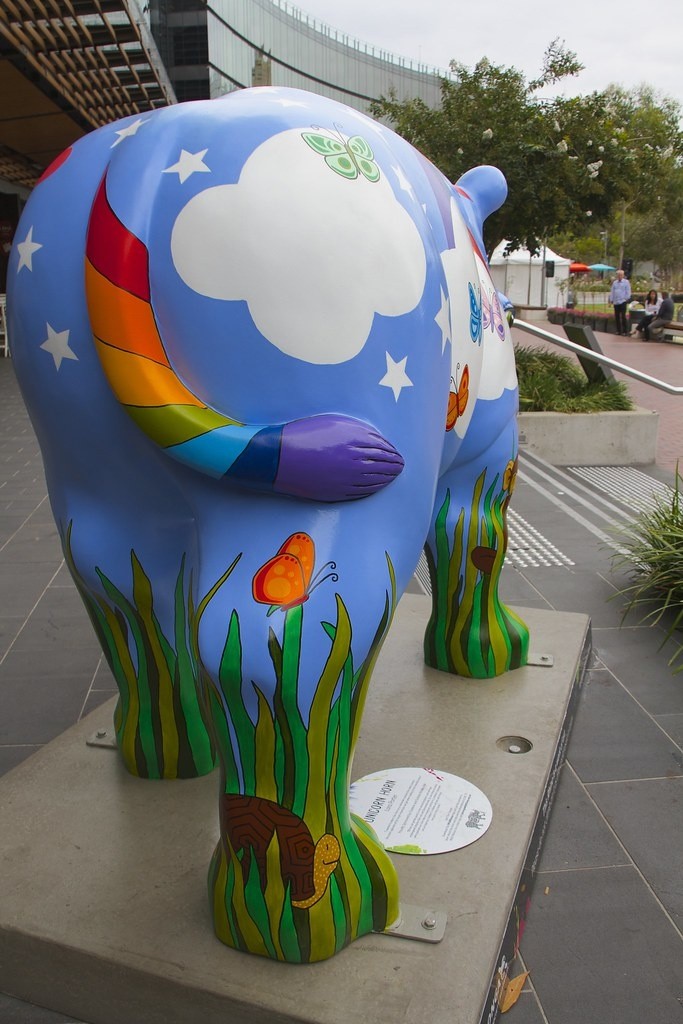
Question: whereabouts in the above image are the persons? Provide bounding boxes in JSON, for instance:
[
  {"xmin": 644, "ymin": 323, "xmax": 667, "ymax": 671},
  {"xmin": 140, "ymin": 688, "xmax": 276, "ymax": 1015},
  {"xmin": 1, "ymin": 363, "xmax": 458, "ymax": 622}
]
[
  {"xmin": 631, "ymin": 290, "xmax": 663, "ymax": 338},
  {"xmin": 642, "ymin": 290, "xmax": 674, "ymax": 342},
  {"xmin": 608, "ymin": 270, "xmax": 632, "ymax": 336}
]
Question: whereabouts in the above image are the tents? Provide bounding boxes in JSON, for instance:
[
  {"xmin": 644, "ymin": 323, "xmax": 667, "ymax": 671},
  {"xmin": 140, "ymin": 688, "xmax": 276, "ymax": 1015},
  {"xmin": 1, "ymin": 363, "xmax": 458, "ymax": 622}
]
[{"xmin": 488, "ymin": 238, "xmax": 572, "ymax": 308}]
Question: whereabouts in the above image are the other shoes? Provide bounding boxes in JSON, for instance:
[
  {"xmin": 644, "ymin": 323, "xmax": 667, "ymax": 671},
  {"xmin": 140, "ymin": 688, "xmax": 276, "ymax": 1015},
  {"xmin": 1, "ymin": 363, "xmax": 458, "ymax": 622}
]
[
  {"xmin": 623, "ymin": 333, "xmax": 626, "ymax": 336},
  {"xmin": 643, "ymin": 338, "xmax": 647, "ymax": 341},
  {"xmin": 630, "ymin": 329, "xmax": 638, "ymax": 334},
  {"xmin": 616, "ymin": 331, "xmax": 619, "ymax": 334}
]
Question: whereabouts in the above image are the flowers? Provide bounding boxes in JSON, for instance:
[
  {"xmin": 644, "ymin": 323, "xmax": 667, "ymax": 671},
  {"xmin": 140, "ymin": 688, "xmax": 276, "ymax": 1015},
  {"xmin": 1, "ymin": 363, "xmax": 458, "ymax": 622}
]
[
  {"xmin": 609, "ymin": 314, "xmax": 629, "ymax": 323},
  {"xmin": 568, "ymin": 309, "xmax": 578, "ymax": 315},
  {"xmin": 574, "ymin": 311, "xmax": 586, "ymax": 318},
  {"xmin": 595, "ymin": 313, "xmax": 611, "ymax": 321},
  {"xmin": 547, "ymin": 307, "xmax": 557, "ymax": 315},
  {"xmin": 584, "ymin": 311, "xmax": 598, "ymax": 319},
  {"xmin": 556, "ymin": 308, "xmax": 566, "ymax": 315}
]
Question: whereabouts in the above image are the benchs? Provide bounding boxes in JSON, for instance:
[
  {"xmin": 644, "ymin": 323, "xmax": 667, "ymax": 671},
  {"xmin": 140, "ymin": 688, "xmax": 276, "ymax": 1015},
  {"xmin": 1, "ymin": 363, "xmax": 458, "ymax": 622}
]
[{"xmin": 649, "ymin": 322, "xmax": 683, "ymax": 342}]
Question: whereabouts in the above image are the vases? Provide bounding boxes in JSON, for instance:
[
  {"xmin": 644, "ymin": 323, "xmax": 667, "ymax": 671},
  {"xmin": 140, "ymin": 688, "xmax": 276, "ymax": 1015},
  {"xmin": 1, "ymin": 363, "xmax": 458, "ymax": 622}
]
[
  {"xmin": 574, "ymin": 317, "xmax": 584, "ymax": 325},
  {"xmin": 595, "ymin": 321, "xmax": 608, "ymax": 333},
  {"xmin": 555, "ymin": 315, "xmax": 565, "ymax": 325},
  {"xmin": 606, "ymin": 322, "xmax": 631, "ymax": 337},
  {"xmin": 584, "ymin": 318, "xmax": 595, "ymax": 331},
  {"xmin": 548, "ymin": 314, "xmax": 555, "ymax": 324},
  {"xmin": 564, "ymin": 315, "xmax": 574, "ymax": 325}
]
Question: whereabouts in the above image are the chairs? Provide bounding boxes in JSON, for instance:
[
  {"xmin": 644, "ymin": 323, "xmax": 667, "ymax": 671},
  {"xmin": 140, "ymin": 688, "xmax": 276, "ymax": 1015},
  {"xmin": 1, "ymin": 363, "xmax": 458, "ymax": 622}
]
[{"xmin": 0, "ymin": 294, "xmax": 11, "ymax": 358}]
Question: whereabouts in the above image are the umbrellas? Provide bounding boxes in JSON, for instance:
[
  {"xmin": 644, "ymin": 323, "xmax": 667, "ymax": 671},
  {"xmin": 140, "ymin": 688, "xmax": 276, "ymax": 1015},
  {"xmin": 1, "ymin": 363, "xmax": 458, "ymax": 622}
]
[
  {"xmin": 569, "ymin": 263, "xmax": 592, "ymax": 278},
  {"xmin": 587, "ymin": 264, "xmax": 617, "ymax": 280}
]
